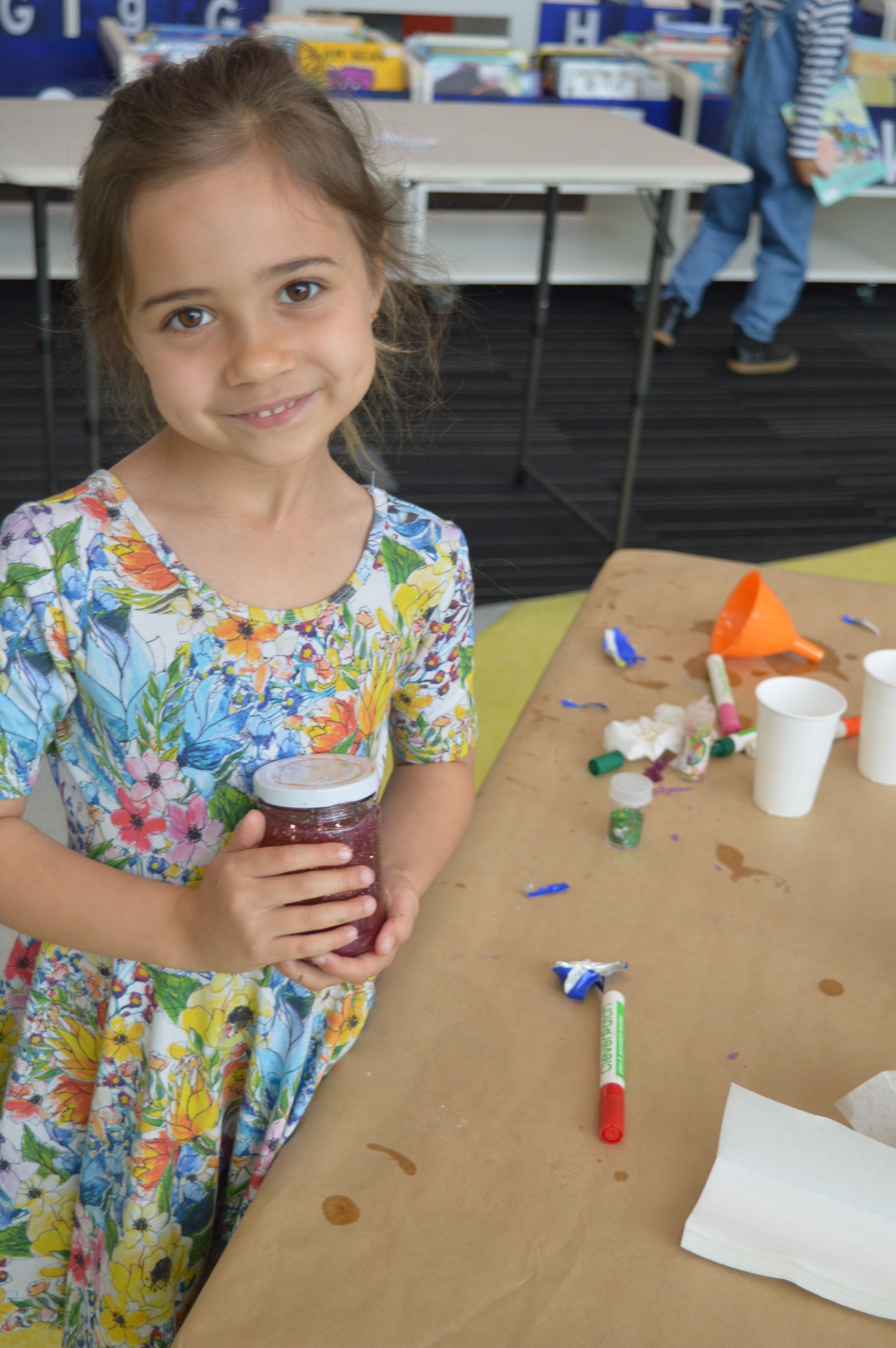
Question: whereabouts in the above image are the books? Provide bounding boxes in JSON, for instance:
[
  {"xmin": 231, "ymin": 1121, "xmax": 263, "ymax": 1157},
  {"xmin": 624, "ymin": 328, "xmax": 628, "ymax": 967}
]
[{"xmin": 780, "ymin": 75, "xmax": 888, "ymax": 208}]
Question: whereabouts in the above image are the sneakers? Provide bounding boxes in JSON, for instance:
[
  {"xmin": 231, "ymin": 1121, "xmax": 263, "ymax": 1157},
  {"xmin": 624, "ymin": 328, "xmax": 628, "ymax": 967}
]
[
  {"xmin": 727, "ymin": 341, "xmax": 798, "ymax": 376},
  {"xmin": 633, "ymin": 311, "xmax": 679, "ymax": 349}
]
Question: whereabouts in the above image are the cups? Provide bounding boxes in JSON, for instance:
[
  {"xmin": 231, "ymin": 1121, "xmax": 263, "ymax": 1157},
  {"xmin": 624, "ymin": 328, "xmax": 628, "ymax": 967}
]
[
  {"xmin": 857, "ymin": 648, "xmax": 896, "ymax": 787},
  {"xmin": 752, "ymin": 675, "xmax": 848, "ymax": 818}
]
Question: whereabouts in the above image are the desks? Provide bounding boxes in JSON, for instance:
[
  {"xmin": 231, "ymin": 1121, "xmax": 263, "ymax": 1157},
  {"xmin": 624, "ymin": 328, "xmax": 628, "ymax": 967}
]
[
  {"xmin": 0, "ymin": 102, "xmax": 752, "ymax": 551},
  {"xmin": 177, "ymin": 550, "xmax": 896, "ymax": 1348}
]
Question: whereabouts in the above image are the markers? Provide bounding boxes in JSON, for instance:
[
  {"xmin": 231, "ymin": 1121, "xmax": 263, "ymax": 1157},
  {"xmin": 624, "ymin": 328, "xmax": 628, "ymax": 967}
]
[
  {"xmin": 600, "ymin": 990, "xmax": 625, "ymax": 1142},
  {"xmin": 709, "ymin": 715, "xmax": 861, "ymax": 758},
  {"xmin": 705, "ymin": 653, "xmax": 740, "ymax": 737}
]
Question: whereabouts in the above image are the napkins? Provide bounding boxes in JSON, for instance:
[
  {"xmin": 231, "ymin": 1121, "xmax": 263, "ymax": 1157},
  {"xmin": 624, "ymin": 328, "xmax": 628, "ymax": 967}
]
[{"xmin": 681, "ymin": 1066, "xmax": 896, "ymax": 1320}]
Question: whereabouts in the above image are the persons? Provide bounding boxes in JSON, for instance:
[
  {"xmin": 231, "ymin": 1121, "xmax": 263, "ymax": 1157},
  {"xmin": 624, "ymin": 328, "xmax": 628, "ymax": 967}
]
[
  {"xmin": 644, "ymin": 0, "xmax": 853, "ymax": 375},
  {"xmin": 0, "ymin": 39, "xmax": 475, "ymax": 1348}
]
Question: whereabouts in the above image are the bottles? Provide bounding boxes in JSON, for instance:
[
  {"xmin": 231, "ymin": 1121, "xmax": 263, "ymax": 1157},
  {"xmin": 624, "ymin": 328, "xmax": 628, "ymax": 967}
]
[{"xmin": 252, "ymin": 753, "xmax": 388, "ymax": 968}]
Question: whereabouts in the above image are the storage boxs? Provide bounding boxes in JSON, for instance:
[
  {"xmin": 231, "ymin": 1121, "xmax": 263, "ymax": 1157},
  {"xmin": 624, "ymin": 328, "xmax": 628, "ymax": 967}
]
[{"xmin": 130, "ymin": 10, "xmax": 676, "ymax": 81}]
